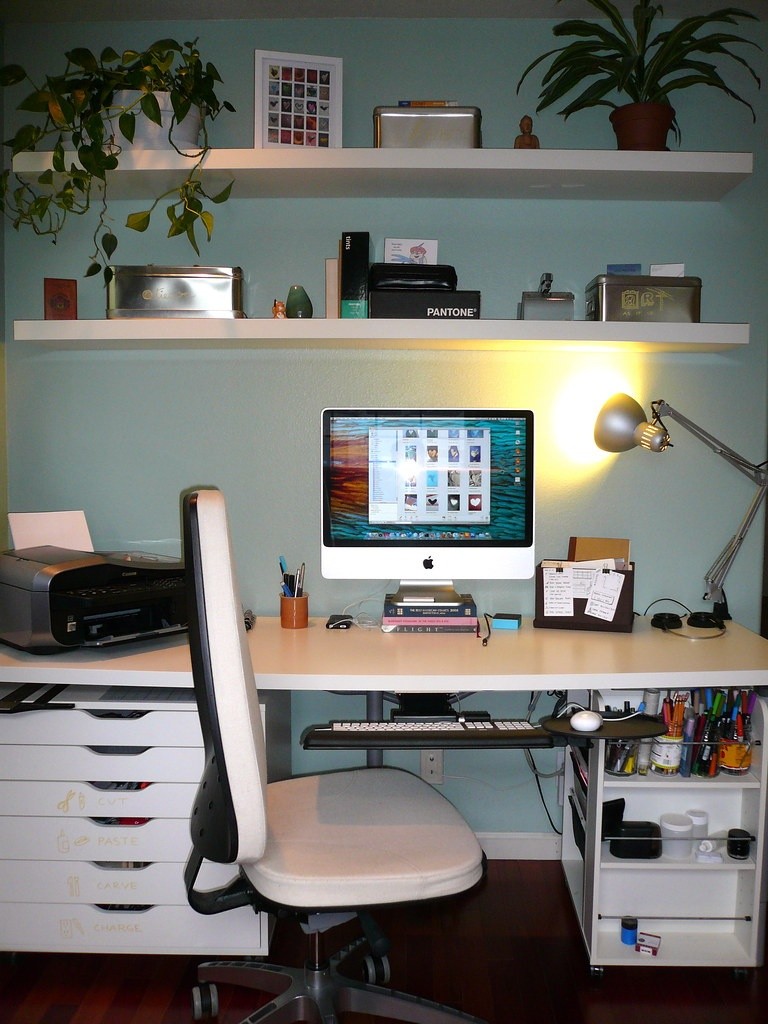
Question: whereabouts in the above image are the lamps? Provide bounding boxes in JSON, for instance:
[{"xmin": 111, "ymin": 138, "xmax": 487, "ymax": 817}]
[{"xmin": 594, "ymin": 392, "xmax": 767, "ymax": 621}]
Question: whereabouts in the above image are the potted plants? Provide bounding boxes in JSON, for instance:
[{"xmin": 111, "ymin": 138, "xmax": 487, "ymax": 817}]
[
  {"xmin": 516, "ymin": 0, "xmax": 762, "ymax": 150},
  {"xmin": 2, "ymin": 36, "xmax": 236, "ymax": 290}
]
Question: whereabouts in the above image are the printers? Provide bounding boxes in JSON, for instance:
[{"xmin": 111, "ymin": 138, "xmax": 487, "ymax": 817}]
[{"xmin": 0, "ymin": 543, "xmax": 190, "ymax": 656}]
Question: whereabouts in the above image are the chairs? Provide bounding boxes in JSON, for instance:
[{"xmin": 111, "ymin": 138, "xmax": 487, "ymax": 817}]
[{"xmin": 182, "ymin": 488, "xmax": 487, "ymax": 1024}]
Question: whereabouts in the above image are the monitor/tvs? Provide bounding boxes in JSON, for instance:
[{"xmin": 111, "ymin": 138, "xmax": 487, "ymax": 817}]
[{"xmin": 320, "ymin": 406, "xmax": 535, "ymax": 607}]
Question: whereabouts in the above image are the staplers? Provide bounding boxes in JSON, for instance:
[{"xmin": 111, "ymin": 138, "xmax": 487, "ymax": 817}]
[{"xmin": 535, "ymin": 273, "xmax": 558, "ymax": 292}]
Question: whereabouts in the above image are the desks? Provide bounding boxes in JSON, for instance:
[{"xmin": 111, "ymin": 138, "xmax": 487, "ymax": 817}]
[{"xmin": 0, "ymin": 615, "xmax": 768, "ymax": 770}]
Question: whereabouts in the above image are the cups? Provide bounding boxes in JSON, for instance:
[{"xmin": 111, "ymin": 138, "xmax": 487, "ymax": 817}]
[
  {"xmin": 720, "ymin": 737, "xmax": 753, "ymax": 777},
  {"xmin": 279, "ymin": 592, "xmax": 309, "ymax": 629},
  {"xmin": 604, "ymin": 739, "xmax": 640, "ymax": 777},
  {"xmin": 648, "ymin": 735, "xmax": 685, "ymax": 778}
]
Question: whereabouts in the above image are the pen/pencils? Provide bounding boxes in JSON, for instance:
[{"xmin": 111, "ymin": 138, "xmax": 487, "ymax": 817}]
[
  {"xmin": 605, "ymin": 696, "xmax": 646, "ymax": 774},
  {"xmin": 662, "ymin": 694, "xmax": 685, "ymax": 741},
  {"xmin": 695, "ymin": 689, "xmax": 723, "ymax": 777},
  {"xmin": 276, "ymin": 556, "xmax": 307, "ymax": 598},
  {"xmin": 727, "ymin": 690, "xmax": 758, "ymax": 741}
]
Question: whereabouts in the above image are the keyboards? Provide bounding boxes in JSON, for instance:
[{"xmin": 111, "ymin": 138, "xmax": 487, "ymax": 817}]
[{"xmin": 332, "ymin": 721, "xmax": 535, "ymax": 731}]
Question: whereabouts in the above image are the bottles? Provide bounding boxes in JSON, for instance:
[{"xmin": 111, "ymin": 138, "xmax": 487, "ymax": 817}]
[
  {"xmin": 621, "ymin": 915, "xmax": 638, "ymax": 945},
  {"xmin": 660, "ymin": 813, "xmax": 692, "ymax": 858},
  {"xmin": 687, "ymin": 809, "xmax": 708, "ymax": 853},
  {"xmin": 698, "ymin": 829, "xmax": 728, "ymax": 852},
  {"xmin": 728, "ymin": 829, "xmax": 751, "ymax": 860}
]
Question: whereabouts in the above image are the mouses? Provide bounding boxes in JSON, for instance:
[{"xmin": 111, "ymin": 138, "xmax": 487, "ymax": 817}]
[{"xmin": 570, "ymin": 711, "xmax": 604, "ymax": 732}]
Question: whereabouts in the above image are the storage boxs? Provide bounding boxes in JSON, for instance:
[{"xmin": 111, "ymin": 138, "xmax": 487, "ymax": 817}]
[
  {"xmin": 105, "ymin": 265, "xmax": 244, "ymax": 319},
  {"xmin": 372, "ymin": 106, "xmax": 484, "ymax": 149},
  {"xmin": 368, "ymin": 290, "xmax": 482, "ymax": 319},
  {"xmin": 635, "ymin": 932, "xmax": 661, "ymax": 956},
  {"xmin": 585, "ymin": 274, "xmax": 702, "ymax": 323}
]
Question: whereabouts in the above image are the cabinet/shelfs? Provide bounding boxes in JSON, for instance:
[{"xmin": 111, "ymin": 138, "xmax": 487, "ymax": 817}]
[
  {"xmin": 11, "ymin": 149, "xmax": 756, "ymax": 350},
  {"xmin": 0, "ymin": 683, "xmax": 273, "ymax": 956},
  {"xmin": 560, "ymin": 689, "xmax": 768, "ymax": 999}
]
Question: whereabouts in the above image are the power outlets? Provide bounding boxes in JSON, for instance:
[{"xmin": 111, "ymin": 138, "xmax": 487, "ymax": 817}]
[{"xmin": 420, "ymin": 749, "xmax": 445, "ymax": 785}]
